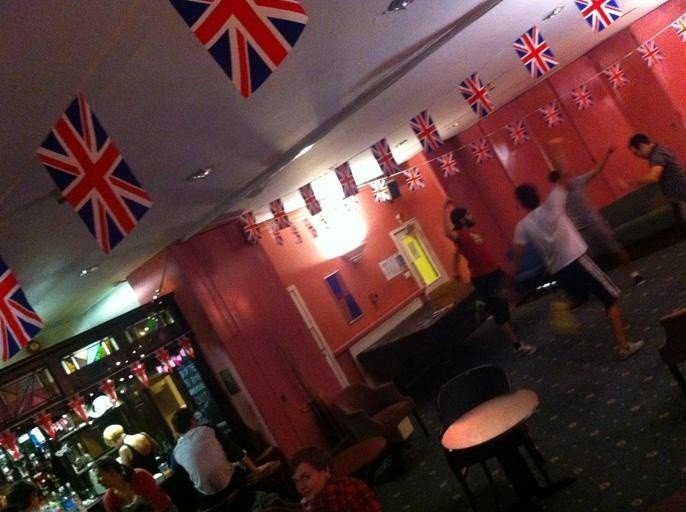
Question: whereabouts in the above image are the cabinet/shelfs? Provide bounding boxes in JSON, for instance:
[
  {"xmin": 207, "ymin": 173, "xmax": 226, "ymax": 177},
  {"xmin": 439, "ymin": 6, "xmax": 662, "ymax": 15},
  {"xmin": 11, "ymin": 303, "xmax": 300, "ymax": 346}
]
[{"xmin": 58, "ymin": 407, "xmax": 131, "ymax": 476}]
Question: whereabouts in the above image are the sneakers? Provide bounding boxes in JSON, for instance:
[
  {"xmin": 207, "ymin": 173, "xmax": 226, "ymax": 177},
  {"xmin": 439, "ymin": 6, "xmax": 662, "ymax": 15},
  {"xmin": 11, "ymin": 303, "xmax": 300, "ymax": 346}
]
[
  {"xmin": 614, "ymin": 340, "xmax": 644, "ymax": 360},
  {"xmin": 475, "ymin": 301, "xmax": 486, "ymax": 326},
  {"xmin": 547, "ymin": 303, "xmax": 569, "ymax": 337},
  {"xmin": 514, "ymin": 344, "xmax": 537, "ymax": 356},
  {"xmin": 632, "ymin": 275, "xmax": 644, "ymax": 288}
]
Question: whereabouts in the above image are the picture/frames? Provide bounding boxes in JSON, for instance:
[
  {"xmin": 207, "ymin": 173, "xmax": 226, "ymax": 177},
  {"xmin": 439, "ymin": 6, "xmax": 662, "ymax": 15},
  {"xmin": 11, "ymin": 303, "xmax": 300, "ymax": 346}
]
[{"xmin": 321, "ymin": 269, "xmax": 364, "ymax": 326}]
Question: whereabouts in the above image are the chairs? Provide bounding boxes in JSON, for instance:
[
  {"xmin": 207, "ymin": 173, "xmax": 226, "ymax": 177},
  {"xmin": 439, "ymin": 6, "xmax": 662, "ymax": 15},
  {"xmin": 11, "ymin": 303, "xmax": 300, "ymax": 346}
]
[
  {"xmin": 331, "ymin": 383, "xmax": 431, "ymax": 466},
  {"xmin": 658, "ymin": 307, "xmax": 686, "ymax": 391},
  {"xmin": 434, "ymin": 363, "xmax": 551, "ymax": 512}
]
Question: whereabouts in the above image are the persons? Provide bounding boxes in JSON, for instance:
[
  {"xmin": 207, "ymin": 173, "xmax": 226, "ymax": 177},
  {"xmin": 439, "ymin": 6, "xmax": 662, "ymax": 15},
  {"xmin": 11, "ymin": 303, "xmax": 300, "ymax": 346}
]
[
  {"xmin": 441, "ymin": 197, "xmax": 539, "ymax": 358},
  {"xmin": 92, "ymin": 456, "xmax": 179, "ymax": 511},
  {"xmin": 101, "ymin": 424, "xmax": 160, "ymax": 476},
  {"xmin": 501, "ymin": 135, "xmax": 647, "ymax": 362},
  {"xmin": 624, "ymin": 132, "xmax": 686, "ymax": 238},
  {"xmin": 286, "ymin": 444, "xmax": 385, "ymax": 511},
  {"xmin": 545, "ymin": 143, "xmax": 644, "ymax": 286},
  {"xmin": 0, "ymin": 477, "xmax": 41, "ymax": 511},
  {"xmin": 169, "ymin": 407, "xmax": 264, "ymax": 511}
]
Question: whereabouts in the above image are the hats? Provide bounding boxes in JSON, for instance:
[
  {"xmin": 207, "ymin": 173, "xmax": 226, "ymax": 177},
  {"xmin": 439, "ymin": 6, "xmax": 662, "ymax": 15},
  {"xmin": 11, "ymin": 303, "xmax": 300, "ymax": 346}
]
[{"xmin": 451, "ymin": 208, "xmax": 467, "ymax": 230}]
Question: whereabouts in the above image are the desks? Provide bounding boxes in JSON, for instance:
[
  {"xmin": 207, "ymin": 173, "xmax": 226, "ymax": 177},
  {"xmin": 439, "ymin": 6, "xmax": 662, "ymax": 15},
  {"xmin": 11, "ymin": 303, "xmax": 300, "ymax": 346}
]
[
  {"xmin": 440, "ymin": 388, "xmax": 543, "ymax": 512},
  {"xmin": 357, "ymin": 277, "xmax": 484, "ymax": 438},
  {"xmin": 328, "ymin": 435, "xmax": 387, "ymax": 478}
]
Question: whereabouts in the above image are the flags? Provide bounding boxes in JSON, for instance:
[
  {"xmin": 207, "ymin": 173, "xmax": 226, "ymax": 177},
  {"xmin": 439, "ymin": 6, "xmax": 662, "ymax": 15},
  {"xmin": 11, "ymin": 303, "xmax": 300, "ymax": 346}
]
[
  {"xmin": 458, "ymin": 70, "xmax": 495, "ymax": 123},
  {"xmin": 238, "ymin": 137, "xmax": 426, "ymax": 249},
  {"xmin": 505, "ymin": 118, "xmax": 532, "ymax": 146},
  {"xmin": 0, "ymin": 250, "xmax": 44, "ymax": 366},
  {"xmin": 603, "ymin": 62, "xmax": 631, "ymax": 92},
  {"xmin": 409, "ymin": 109, "xmax": 445, "ymax": 155},
  {"xmin": 511, "ymin": 24, "xmax": 559, "ymax": 81},
  {"xmin": 167, "ymin": 1, "xmax": 309, "ymax": 101},
  {"xmin": 436, "ymin": 151, "xmax": 463, "ymax": 181},
  {"xmin": 569, "ymin": 83, "xmax": 595, "ymax": 113},
  {"xmin": 34, "ymin": 88, "xmax": 155, "ymax": 257},
  {"xmin": 670, "ymin": 13, "xmax": 686, "ymax": 45},
  {"xmin": 635, "ymin": 38, "xmax": 666, "ymax": 69},
  {"xmin": 538, "ymin": 100, "xmax": 563, "ymax": 131},
  {"xmin": 574, "ymin": 0, "xmax": 624, "ymax": 35},
  {"xmin": 468, "ymin": 137, "xmax": 494, "ymax": 165}
]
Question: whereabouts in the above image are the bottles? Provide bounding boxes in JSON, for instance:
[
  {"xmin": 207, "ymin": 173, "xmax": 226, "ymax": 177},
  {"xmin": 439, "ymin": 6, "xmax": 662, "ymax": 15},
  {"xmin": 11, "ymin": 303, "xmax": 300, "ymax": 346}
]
[{"xmin": 50, "ymin": 482, "xmax": 87, "ymax": 512}]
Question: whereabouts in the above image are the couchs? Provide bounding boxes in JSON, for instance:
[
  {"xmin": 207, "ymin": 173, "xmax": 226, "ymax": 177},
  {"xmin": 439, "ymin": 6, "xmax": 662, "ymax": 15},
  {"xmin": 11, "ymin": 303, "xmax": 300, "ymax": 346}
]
[{"xmin": 506, "ymin": 181, "xmax": 686, "ymax": 307}]
